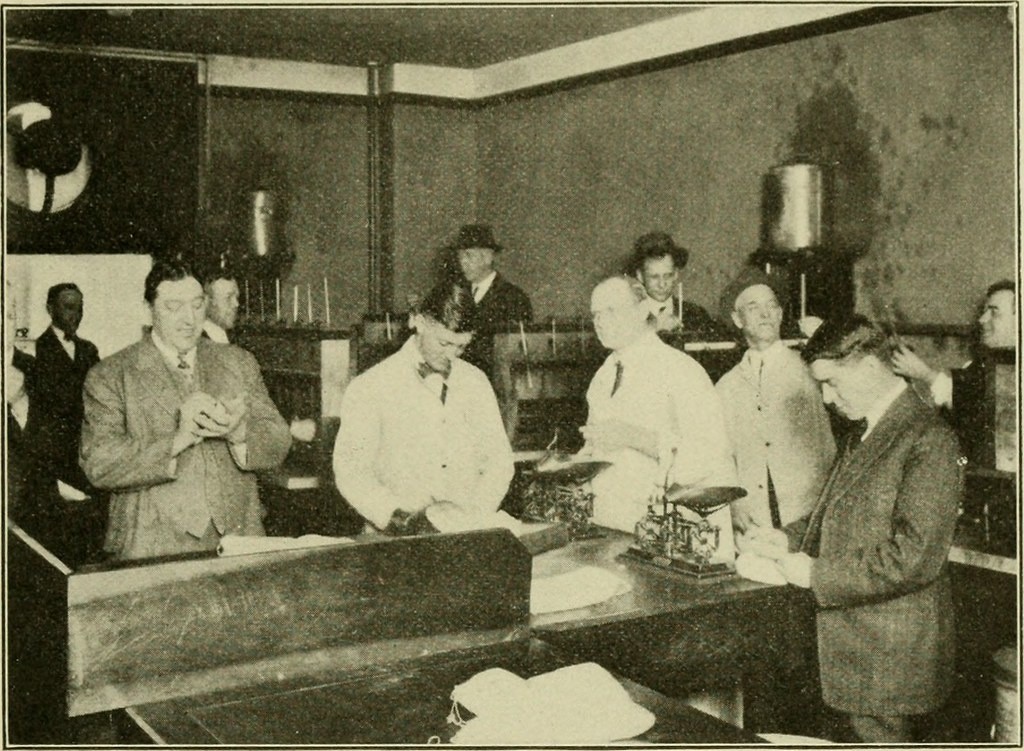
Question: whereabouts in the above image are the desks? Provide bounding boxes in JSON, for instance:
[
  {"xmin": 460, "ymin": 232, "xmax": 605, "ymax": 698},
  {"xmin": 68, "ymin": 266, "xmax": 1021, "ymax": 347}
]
[{"xmin": 117, "ymin": 517, "xmax": 780, "ymax": 746}]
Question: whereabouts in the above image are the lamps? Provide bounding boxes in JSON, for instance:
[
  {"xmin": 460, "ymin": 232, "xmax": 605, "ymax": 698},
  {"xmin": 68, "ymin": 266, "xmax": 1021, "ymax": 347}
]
[
  {"xmin": 764, "ymin": 155, "xmax": 823, "ymax": 336},
  {"xmin": 232, "ymin": 187, "xmax": 277, "ymax": 333}
]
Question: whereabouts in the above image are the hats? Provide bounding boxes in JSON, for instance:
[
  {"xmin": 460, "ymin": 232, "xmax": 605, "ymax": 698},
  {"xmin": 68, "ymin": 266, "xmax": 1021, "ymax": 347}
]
[
  {"xmin": 448, "ymin": 225, "xmax": 504, "ymax": 251},
  {"xmin": 626, "ymin": 233, "xmax": 688, "ymax": 276}
]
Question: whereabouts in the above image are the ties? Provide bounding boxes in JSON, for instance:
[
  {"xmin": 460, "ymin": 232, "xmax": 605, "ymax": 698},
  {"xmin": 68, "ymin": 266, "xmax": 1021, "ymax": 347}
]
[
  {"xmin": 612, "ymin": 360, "xmax": 623, "ymax": 398},
  {"xmin": 177, "ymin": 353, "xmax": 188, "ymax": 371}
]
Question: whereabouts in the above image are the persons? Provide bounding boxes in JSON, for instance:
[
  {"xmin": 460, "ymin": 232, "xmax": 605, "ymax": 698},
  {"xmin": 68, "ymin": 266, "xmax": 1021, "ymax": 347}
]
[
  {"xmin": 892, "ymin": 280, "xmax": 1015, "ymax": 411},
  {"xmin": 80, "ymin": 259, "xmax": 290, "ymax": 562},
  {"xmin": 333, "ymin": 284, "xmax": 516, "ymax": 536},
  {"xmin": 714, "ymin": 285, "xmax": 839, "ymax": 535},
  {"xmin": 746, "ymin": 314, "xmax": 962, "ymax": 744},
  {"xmin": 398, "ymin": 226, "xmax": 533, "ymax": 372},
  {"xmin": 636, "ymin": 232, "xmax": 718, "ymax": 386},
  {"xmin": 201, "ymin": 267, "xmax": 241, "ymax": 343},
  {"xmin": 24, "ymin": 282, "xmax": 101, "ymax": 483},
  {"xmin": 579, "ymin": 276, "xmax": 736, "ymax": 560}
]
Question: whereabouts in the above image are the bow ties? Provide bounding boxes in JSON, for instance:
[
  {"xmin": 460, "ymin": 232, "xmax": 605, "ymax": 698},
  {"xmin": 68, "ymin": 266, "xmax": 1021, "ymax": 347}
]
[
  {"xmin": 64, "ymin": 333, "xmax": 78, "ymax": 343},
  {"xmin": 419, "ymin": 361, "xmax": 453, "ymax": 380}
]
